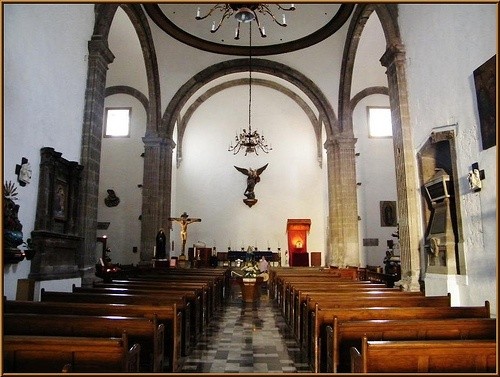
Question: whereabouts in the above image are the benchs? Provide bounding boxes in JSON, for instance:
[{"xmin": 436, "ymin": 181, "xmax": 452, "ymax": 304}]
[
  {"xmin": 269, "ymin": 266, "xmax": 496, "ymax": 373},
  {"xmin": 3, "ymin": 264, "xmax": 231, "ymax": 373}
]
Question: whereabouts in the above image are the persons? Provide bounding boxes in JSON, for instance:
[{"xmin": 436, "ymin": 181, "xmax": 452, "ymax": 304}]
[
  {"xmin": 258, "ymin": 255, "xmax": 269, "ymax": 272},
  {"xmin": 383, "ymin": 250, "xmax": 401, "ymax": 277},
  {"xmin": 174, "ymin": 219, "xmax": 197, "ymax": 243},
  {"xmin": 155, "ymin": 229, "xmax": 167, "ymax": 258}
]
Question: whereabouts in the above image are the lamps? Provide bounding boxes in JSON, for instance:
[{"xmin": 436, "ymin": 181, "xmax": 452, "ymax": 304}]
[{"xmin": 195, "ymin": 3, "xmax": 296, "ymax": 155}]
